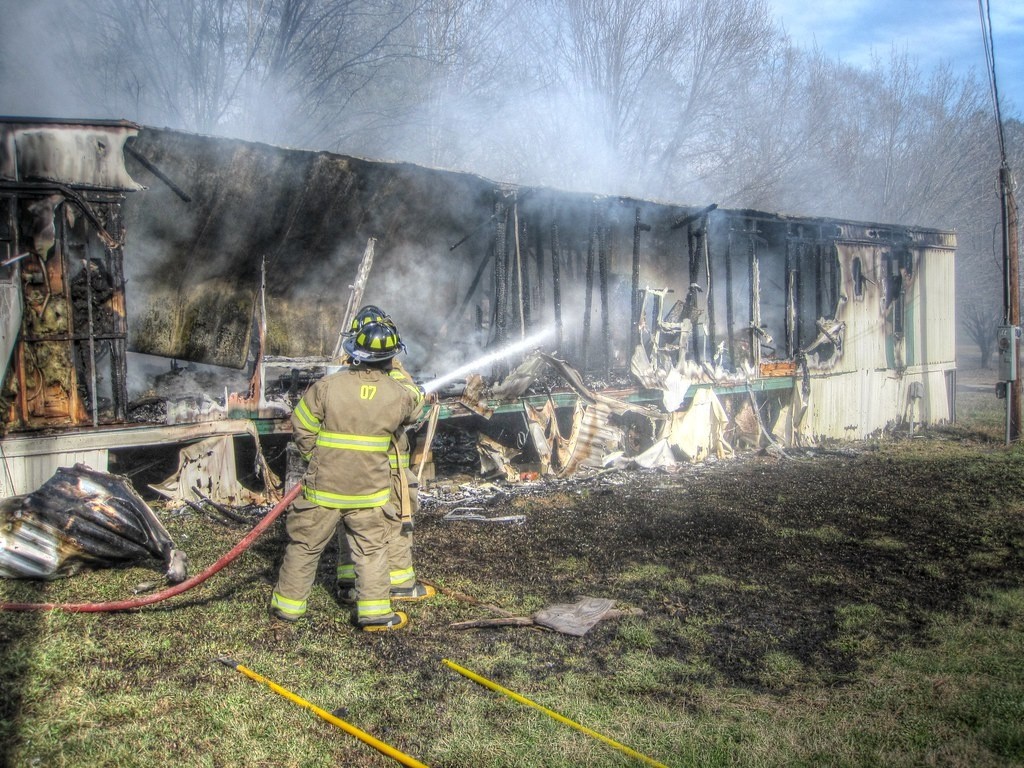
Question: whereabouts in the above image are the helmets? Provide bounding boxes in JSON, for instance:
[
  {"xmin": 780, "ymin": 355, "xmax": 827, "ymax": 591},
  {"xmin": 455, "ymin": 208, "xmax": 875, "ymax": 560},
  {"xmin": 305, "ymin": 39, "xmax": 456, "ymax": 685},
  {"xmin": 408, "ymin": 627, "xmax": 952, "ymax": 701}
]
[
  {"xmin": 344, "ymin": 322, "xmax": 404, "ymax": 362},
  {"xmin": 339, "ymin": 305, "xmax": 389, "ymax": 338}
]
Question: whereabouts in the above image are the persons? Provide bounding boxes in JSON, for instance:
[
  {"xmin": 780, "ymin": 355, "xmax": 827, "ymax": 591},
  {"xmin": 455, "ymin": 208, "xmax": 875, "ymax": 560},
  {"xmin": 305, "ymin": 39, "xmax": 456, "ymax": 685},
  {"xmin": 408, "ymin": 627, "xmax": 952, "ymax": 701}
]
[
  {"xmin": 336, "ymin": 305, "xmax": 435, "ymax": 606},
  {"xmin": 270, "ymin": 322, "xmax": 423, "ymax": 633}
]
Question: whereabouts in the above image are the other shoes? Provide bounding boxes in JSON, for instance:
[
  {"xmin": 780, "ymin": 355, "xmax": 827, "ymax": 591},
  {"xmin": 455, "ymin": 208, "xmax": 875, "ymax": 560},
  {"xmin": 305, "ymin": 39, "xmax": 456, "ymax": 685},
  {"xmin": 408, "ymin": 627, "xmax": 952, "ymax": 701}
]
[
  {"xmin": 363, "ymin": 612, "xmax": 406, "ymax": 631},
  {"xmin": 276, "ymin": 610, "xmax": 299, "ymax": 621},
  {"xmin": 339, "ymin": 585, "xmax": 357, "ymax": 602},
  {"xmin": 389, "ymin": 585, "xmax": 436, "ymax": 601}
]
